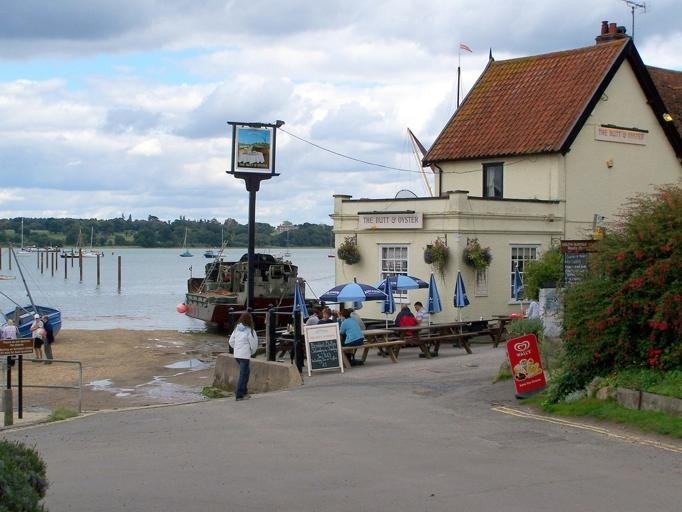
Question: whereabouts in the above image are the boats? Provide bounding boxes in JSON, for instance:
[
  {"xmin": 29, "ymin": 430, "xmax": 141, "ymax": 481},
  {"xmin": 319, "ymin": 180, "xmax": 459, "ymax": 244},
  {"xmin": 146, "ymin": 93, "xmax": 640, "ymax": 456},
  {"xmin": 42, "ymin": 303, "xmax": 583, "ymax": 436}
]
[
  {"xmin": 182, "ymin": 245, "xmax": 326, "ymax": 330},
  {"xmin": 203, "ymin": 249, "xmax": 218, "ymax": 257}
]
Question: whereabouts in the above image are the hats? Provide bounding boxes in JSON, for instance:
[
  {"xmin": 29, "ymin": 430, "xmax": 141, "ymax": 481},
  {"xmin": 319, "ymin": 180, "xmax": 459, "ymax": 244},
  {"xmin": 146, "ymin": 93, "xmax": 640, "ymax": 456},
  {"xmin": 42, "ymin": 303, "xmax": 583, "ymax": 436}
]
[{"xmin": 34, "ymin": 314, "xmax": 39, "ymax": 318}]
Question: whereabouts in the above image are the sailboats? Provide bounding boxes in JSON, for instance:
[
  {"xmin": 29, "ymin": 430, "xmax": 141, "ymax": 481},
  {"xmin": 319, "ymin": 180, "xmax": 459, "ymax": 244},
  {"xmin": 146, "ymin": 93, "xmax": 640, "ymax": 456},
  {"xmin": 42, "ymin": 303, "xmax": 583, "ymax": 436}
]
[
  {"xmin": 219, "ymin": 225, "xmax": 228, "ymax": 257},
  {"xmin": 0, "ymin": 231, "xmax": 63, "ymax": 343},
  {"xmin": 14, "ymin": 216, "xmax": 104, "ymax": 258},
  {"xmin": 178, "ymin": 227, "xmax": 194, "ymax": 257}
]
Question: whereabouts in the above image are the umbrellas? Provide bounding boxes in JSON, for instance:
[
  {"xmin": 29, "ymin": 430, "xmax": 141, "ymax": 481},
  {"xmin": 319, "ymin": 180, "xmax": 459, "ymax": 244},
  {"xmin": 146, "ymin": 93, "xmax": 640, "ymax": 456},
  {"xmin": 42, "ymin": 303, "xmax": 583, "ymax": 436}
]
[
  {"xmin": 453, "ymin": 270, "xmax": 470, "ymax": 335},
  {"xmin": 512, "ymin": 263, "xmax": 525, "ymax": 313},
  {"xmin": 291, "ymin": 273, "xmax": 442, "ymax": 340}
]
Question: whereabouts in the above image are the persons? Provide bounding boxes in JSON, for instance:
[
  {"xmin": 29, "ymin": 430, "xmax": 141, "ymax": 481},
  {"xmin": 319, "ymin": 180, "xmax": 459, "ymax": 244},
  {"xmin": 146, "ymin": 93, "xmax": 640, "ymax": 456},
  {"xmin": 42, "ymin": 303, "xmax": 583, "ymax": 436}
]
[
  {"xmin": 305, "ymin": 306, "xmax": 366, "ymax": 360},
  {"xmin": 30, "ymin": 314, "xmax": 53, "ymax": 364},
  {"xmin": 395, "ymin": 301, "xmax": 429, "ymax": 356},
  {"xmin": 2, "ymin": 318, "xmax": 20, "ymax": 367},
  {"xmin": 228, "ymin": 312, "xmax": 259, "ymax": 401},
  {"xmin": 527, "ymin": 295, "xmax": 539, "ymax": 320}
]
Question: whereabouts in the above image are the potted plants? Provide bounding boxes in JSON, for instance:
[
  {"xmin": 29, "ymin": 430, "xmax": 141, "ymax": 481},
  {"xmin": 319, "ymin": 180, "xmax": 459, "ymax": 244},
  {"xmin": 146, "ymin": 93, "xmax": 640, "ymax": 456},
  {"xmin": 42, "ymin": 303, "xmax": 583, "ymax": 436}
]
[
  {"xmin": 337, "ymin": 236, "xmax": 360, "ymax": 265},
  {"xmin": 463, "ymin": 239, "xmax": 492, "ymax": 273},
  {"xmin": 424, "ymin": 236, "xmax": 449, "ymax": 273}
]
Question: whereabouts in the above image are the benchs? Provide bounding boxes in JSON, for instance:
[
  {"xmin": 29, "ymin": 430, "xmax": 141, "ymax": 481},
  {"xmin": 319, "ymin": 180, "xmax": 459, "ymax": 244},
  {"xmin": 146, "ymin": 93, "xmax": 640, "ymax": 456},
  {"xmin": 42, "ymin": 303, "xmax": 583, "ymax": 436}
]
[{"xmin": 257, "ymin": 326, "xmax": 509, "ymax": 368}]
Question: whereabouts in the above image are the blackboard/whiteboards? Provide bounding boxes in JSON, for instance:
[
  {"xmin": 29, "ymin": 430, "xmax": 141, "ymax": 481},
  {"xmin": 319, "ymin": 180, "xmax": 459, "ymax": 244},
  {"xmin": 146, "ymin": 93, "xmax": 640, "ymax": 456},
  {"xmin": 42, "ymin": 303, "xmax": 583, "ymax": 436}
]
[{"xmin": 304, "ymin": 322, "xmax": 343, "ymax": 371}]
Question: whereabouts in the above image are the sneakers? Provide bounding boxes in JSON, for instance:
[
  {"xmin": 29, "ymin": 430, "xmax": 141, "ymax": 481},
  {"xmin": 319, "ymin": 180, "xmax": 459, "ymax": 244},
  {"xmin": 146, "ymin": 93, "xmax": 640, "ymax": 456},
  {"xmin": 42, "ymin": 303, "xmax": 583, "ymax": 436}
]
[{"xmin": 236, "ymin": 394, "xmax": 251, "ymax": 401}]
